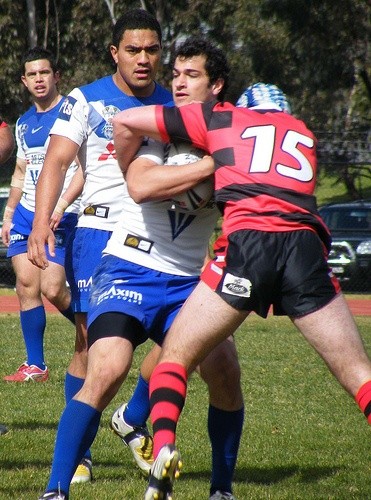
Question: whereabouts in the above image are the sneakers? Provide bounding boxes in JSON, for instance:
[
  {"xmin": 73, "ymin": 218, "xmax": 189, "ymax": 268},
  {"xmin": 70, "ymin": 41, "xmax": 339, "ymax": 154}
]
[
  {"xmin": 109, "ymin": 402, "xmax": 154, "ymax": 478},
  {"xmin": 3, "ymin": 361, "xmax": 49, "ymax": 383},
  {"xmin": 40, "ymin": 490, "xmax": 68, "ymax": 500},
  {"xmin": 69, "ymin": 458, "xmax": 92, "ymax": 483},
  {"xmin": 145, "ymin": 444, "xmax": 183, "ymax": 500}
]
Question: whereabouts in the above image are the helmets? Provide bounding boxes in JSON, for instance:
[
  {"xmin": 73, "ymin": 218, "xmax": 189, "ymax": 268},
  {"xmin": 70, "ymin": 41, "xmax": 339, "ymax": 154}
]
[{"xmin": 235, "ymin": 82, "xmax": 291, "ymax": 115}]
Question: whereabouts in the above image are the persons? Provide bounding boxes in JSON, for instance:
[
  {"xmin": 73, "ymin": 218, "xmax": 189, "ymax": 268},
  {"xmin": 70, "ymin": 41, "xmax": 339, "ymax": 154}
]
[
  {"xmin": 1, "ymin": 47, "xmax": 87, "ymax": 384},
  {"xmin": 27, "ymin": 9, "xmax": 177, "ymax": 484},
  {"xmin": 40, "ymin": 36, "xmax": 245, "ymax": 500},
  {"xmin": 112, "ymin": 82, "xmax": 371, "ymax": 500}
]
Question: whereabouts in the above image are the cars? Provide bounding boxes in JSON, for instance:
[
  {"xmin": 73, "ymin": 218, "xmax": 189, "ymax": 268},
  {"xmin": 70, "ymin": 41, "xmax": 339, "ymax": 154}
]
[{"xmin": 317, "ymin": 202, "xmax": 371, "ymax": 292}]
